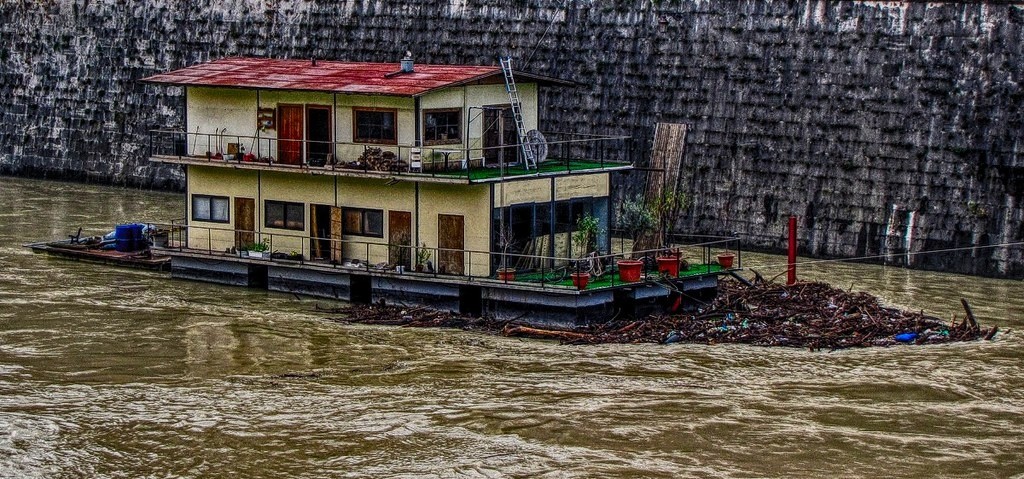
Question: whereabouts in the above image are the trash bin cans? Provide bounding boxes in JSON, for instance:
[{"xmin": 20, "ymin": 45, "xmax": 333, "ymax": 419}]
[
  {"xmin": 115, "ymin": 225, "xmax": 134, "ymax": 251},
  {"xmin": 131, "ymin": 222, "xmax": 145, "ymax": 250}
]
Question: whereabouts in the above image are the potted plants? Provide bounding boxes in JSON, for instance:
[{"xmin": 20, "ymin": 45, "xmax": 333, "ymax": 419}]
[
  {"xmin": 717, "ymin": 207, "xmax": 741, "ymax": 268},
  {"xmin": 236, "ymin": 247, "xmax": 248, "ymax": 257},
  {"xmin": 248, "ymin": 239, "xmax": 270, "ymax": 259},
  {"xmin": 570, "ymin": 213, "xmax": 600, "ymax": 291},
  {"xmin": 415, "ymin": 243, "xmax": 432, "ymax": 273},
  {"xmin": 388, "ymin": 233, "xmax": 409, "ymax": 272},
  {"xmin": 495, "ymin": 224, "xmax": 516, "ymax": 281},
  {"xmin": 616, "ymin": 194, "xmax": 660, "ymax": 282},
  {"xmin": 651, "ymin": 190, "xmax": 687, "ymax": 279}
]
